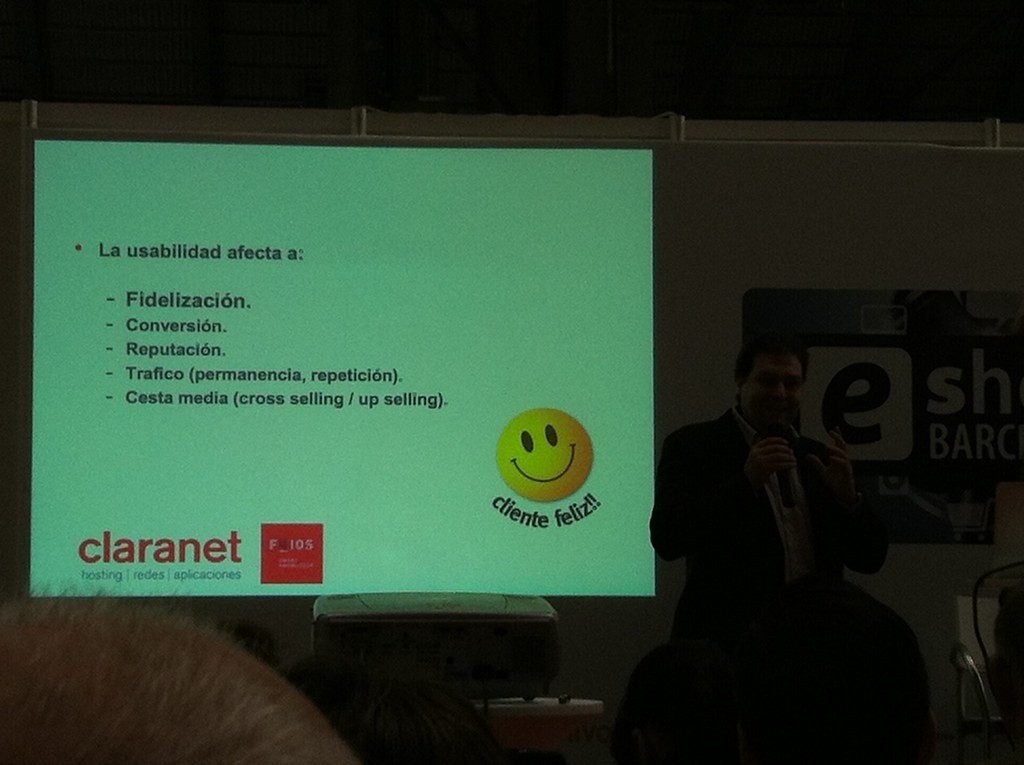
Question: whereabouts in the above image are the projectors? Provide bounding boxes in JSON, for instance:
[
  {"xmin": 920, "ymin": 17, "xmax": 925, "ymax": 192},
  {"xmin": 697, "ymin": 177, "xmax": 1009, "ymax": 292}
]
[{"xmin": 311, "ymin": 593, "xmax": 562, "ymax": 701}]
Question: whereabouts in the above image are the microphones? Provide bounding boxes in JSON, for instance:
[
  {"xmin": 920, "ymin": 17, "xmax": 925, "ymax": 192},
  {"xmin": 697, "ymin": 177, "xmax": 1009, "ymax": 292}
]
[{"xmin": 770, "ymin": 423, "xmax": 799, "ymax": 508}]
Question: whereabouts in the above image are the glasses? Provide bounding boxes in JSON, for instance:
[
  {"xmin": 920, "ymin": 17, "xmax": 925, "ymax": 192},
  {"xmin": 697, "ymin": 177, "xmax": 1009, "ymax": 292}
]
[{"xmin": 753, "ymin": 372, "xmax": 804, "ymax": 389}]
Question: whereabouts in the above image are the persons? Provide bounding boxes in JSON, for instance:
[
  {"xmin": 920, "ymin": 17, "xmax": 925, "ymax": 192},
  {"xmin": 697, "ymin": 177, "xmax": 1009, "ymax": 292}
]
[
  {"xmin": 651, "ymin": 335, "xmax": 855, "ymax": 638},
  {"xmin": 1, "ymin": 585, "xmax": 1024, "ymax": 765}
]
